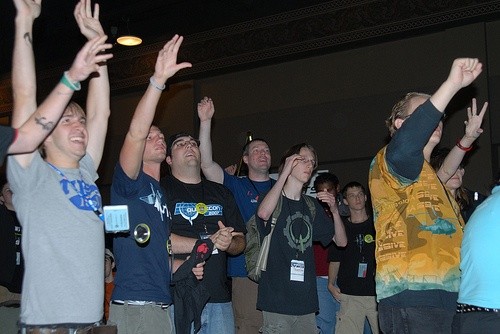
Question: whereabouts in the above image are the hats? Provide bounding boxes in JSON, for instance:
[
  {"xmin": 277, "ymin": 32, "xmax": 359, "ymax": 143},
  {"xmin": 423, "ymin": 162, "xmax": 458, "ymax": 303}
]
[{"xmin": 105, "ymin": 248, "xmax": 117, "ymax": 272}]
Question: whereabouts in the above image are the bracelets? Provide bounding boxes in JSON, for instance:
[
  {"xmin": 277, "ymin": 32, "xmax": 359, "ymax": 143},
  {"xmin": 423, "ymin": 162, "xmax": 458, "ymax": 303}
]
[
  {"xmin": 64, "ymin": 71, "xmax": 82, "ymax": 91},
  {"xmin": 328, "ymin": 206, "xmax": 338, "ymax": 213},
  {"xmin": 455, "ymin": 141, "xmax": 471, "ymax": 152},
  {"xmin": 58, "ymin": 76, "xmax": 78, "ymax": 91},
  {"xmin": 149, "ymin": 76, "xmax": 165, "ymax": 92}
]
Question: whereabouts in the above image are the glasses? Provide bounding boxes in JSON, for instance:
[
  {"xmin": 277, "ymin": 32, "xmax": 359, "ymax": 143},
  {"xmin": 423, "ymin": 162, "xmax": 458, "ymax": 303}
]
[
  {"xmin": 456, "ymin": 168, "xmax": 465, "ymax": 177},
  {"xmin": 295, "ymin": 158, "xmax": 316, "ymax": 167},
  {"xmin": 404, "ymin": 111, "xmax": 447, "ymax": 121},
  {"xmin": 169, "ymin": 138, "xmax": 200, "ymax": 151}
]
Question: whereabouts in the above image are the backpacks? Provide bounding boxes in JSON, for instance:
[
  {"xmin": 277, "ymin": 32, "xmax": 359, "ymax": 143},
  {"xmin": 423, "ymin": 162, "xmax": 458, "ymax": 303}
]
[{"xmin": 245, "ymin": 194, "xmax": 316, "ymax": 283}]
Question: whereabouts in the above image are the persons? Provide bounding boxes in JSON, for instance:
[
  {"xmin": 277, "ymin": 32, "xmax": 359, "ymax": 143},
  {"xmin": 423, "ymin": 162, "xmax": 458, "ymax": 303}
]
[{"xmin": 0, "ymin": 0, "xmax": 500, "ymax": 334}]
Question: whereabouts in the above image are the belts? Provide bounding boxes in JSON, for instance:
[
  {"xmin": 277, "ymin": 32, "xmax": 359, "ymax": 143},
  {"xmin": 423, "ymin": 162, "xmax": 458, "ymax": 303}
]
[
  {"xmin": 455, "ymin": 304, "xmax": 498, "ymax": 312},
  {"xmin": 19, "ymin": 325, "xmax": 117, "ymax": 334}
]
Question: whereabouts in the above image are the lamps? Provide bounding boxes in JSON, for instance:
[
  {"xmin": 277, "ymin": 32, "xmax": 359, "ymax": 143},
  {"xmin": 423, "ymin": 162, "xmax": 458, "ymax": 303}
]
[{"xmin": 115, "ymin": 17, "xmax": 144, "ymax": 47}]
